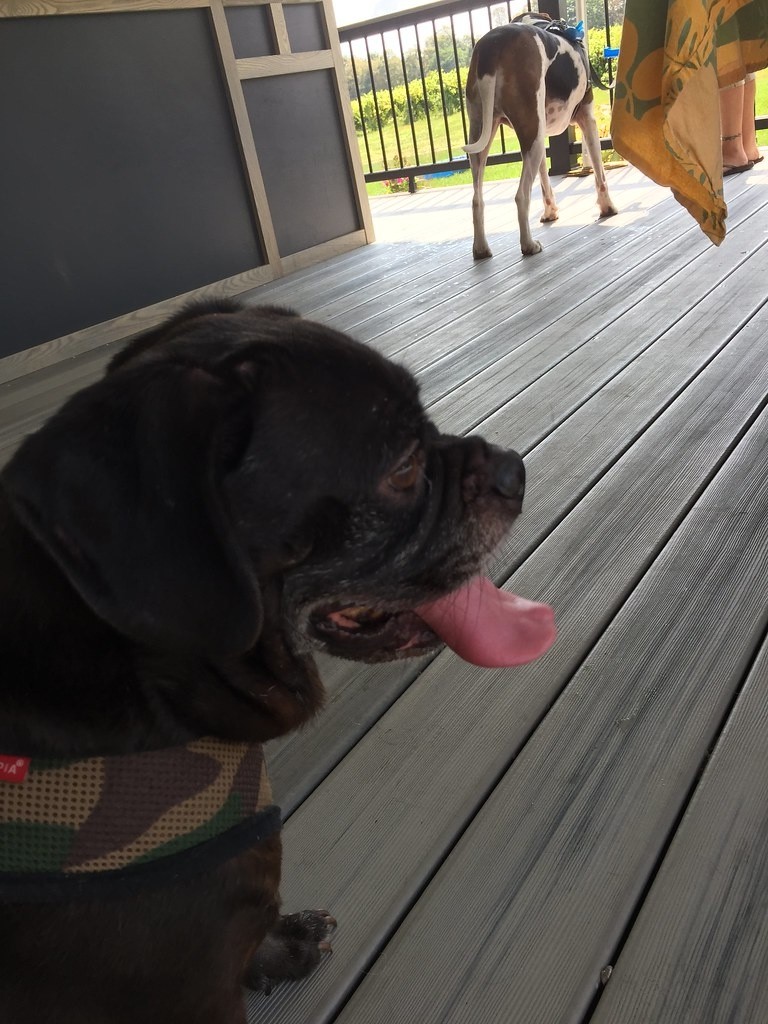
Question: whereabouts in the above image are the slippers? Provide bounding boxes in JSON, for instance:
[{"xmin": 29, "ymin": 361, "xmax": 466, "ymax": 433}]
[{"xmin": 722, "ymin": 154, "xmax": 764, "ymax": 175}]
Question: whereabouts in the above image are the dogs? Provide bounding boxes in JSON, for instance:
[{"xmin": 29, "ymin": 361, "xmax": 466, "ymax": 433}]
[
  {"xmin": 0, "ymin": 296, "xmax": 558, "ymax": 1024},
  {"xmin": 461, "ymin": 12, "xmax": 618, "ymax": 263}
]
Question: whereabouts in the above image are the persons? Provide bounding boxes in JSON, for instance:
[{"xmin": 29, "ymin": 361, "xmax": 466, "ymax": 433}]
[{"xmin": 719, "ymin": 0, "xmax": 768, "ymax": 177}]
